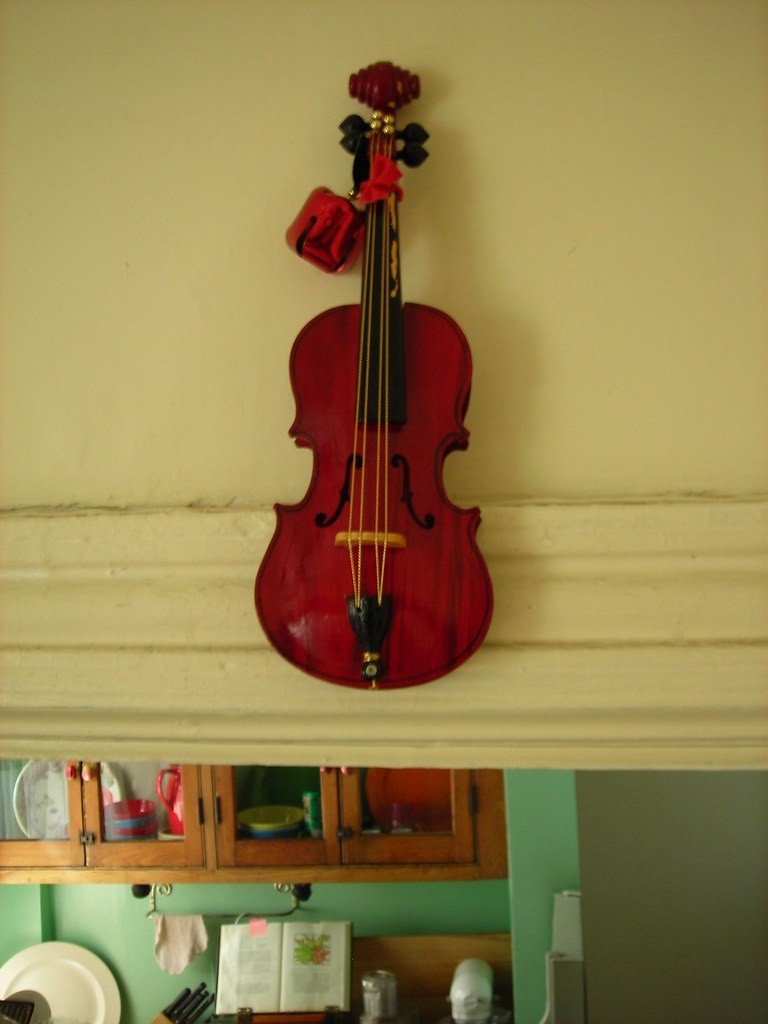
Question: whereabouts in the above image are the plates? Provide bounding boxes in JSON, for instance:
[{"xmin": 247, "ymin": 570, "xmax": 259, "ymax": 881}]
[
  {"xmin": 366, "ymin": 767, "xmax": 454, "ymax": 835},
  {"xmin": 13, "ymin": 761, "xmax": 126, "ymax": 841},
  {"xmin": 0, "ymin": 941, "xmax": 121, "ymax": 1024}
]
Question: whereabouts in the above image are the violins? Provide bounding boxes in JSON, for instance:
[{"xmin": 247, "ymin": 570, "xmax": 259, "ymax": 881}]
[{"xmin": 252, "ymin": 59, "xmax": 495, "ymax": 691}]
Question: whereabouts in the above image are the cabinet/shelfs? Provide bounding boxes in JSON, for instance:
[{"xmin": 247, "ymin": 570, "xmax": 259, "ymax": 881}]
[
  {"xmin": 211, "ymin": 765, "xmax": 506, "ymax": 883},
  {"xmin": 1, "ymin": 759, "xmax": 206, "ymax": 884}
]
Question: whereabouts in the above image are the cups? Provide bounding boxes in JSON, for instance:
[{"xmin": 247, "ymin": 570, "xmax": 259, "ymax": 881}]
[
  {"xmin": 157, "ymin": 764, "xmax": 184, "ymax": 835},
  {"xmin": 102, "ymin": 796, "xmax": 156, "ymax": 841}
]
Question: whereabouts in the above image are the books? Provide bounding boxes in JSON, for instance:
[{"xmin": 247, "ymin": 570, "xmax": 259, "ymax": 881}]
[{"xmin": 213, "ymin": 919, "xmax": 355, "ymax": 1016}]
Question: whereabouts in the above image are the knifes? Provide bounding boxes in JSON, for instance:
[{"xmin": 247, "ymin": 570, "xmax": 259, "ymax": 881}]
[{"xmin": 161, "ymin": 983, "xmax": 219, "ymax": 1024}]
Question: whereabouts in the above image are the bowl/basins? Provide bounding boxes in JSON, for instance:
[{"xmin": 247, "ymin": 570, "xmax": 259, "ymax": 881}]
[
  {"xmin": 237, "ymin": 805, "xmax": 303, "ymax": 840},
  {"xmin": 158, "ymin": 829, "xmax": 183, "ymax": 842}
]
